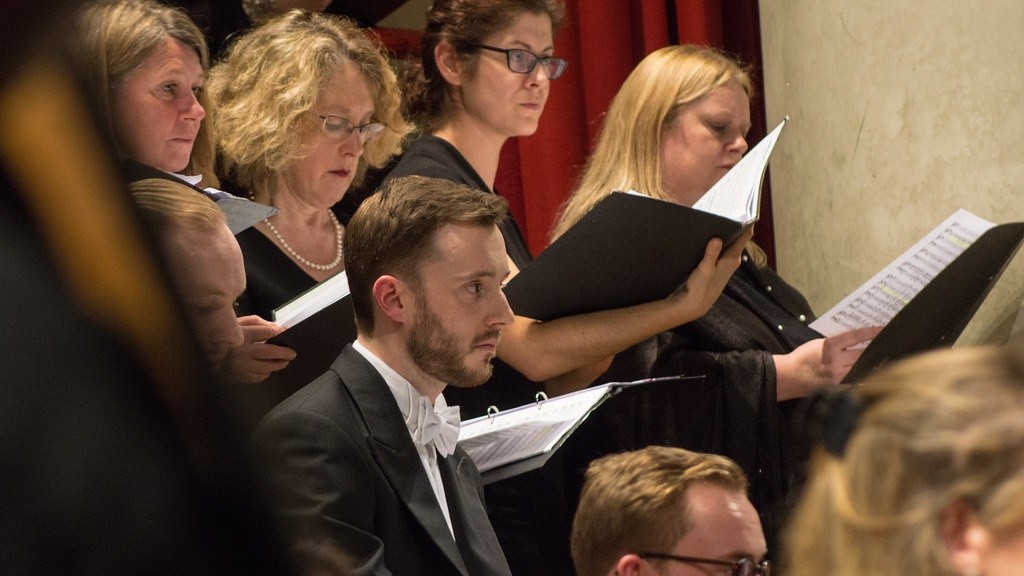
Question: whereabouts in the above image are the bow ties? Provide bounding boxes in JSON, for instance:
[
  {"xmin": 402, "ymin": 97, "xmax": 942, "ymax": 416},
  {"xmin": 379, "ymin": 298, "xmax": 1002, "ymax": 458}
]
[{"xmin": 408, "ymin": 385, "xmax": 464, "ymax": 459}]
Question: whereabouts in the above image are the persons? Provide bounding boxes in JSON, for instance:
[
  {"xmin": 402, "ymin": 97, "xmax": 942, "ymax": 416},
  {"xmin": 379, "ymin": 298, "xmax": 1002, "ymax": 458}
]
[
  {"xmin": 545, "ymin": 45, "xmax": 883, "ymax": 500},
  {"xmin": 198, "ymin": 0, "xmax": 410, "ymax": 63},
  {"xmin": 569, "ymin": 446, "xmax": 770, "ymax": 576},
  {"xmin": 242, "ymin": 175, "xmax": 514, "ymax": 576},
  {"xmin": 46, "ymin": 0, "xmax": 222, "ymax": 204},
  {"xmin": 68, "ymin": 178, "xmax": 247, "ymax": 387},
  {"xmin": 384, "ymin": 0, "xmax": 755, "ymax": 576},
  {"xmin": 205, "ymin": 11, "xmax": 413, "ymax": 334},
  {"xmin": 784, "ymin": 343, "xmax": 1024, "ymax": 576}
]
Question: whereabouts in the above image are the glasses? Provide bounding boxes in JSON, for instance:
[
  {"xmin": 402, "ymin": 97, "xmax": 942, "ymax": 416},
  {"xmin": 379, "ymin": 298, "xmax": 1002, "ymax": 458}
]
[
  {"xmin": 310, "ymin": 111, "xmax": 386, "ymax": 145},
  {"xmin": 610, "ymin": 552, "xmax": 774, "ymax": 576},
  {"xmin": 462, "ymin": 40, "xmax": 568, "ymax": 83}
]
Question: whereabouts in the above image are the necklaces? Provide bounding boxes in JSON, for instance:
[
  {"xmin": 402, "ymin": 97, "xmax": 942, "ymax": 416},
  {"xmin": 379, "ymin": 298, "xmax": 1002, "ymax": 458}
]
[{"xmin": 246, "ymin": 187, "xmax": 343, "ymax": 270}]
